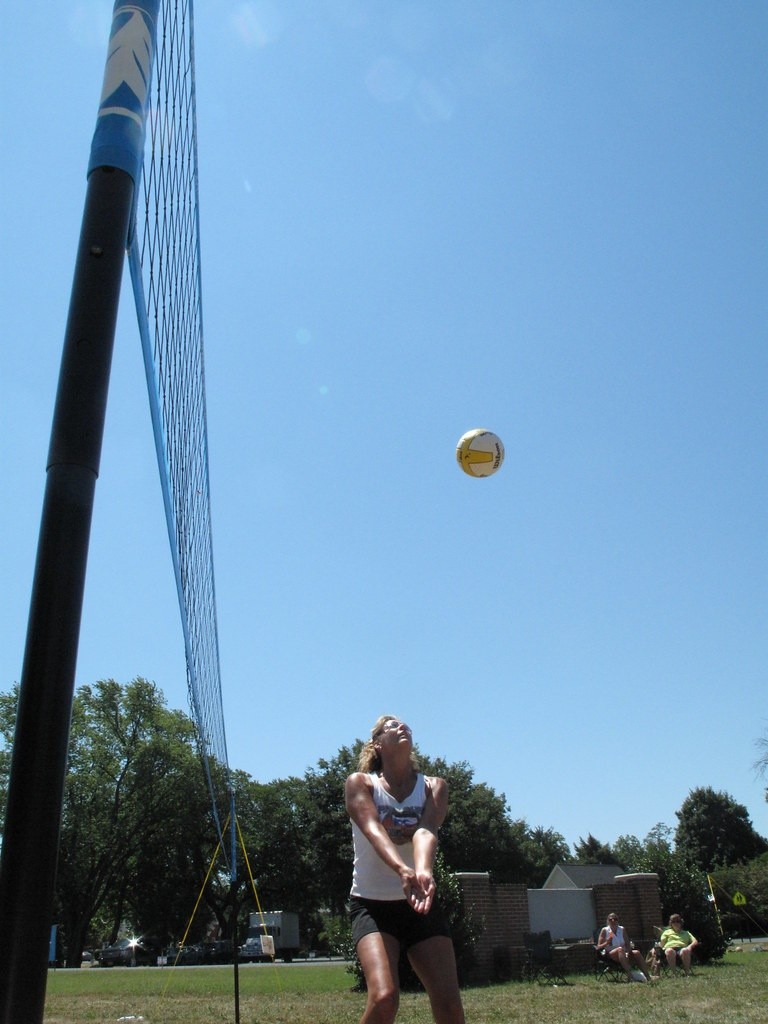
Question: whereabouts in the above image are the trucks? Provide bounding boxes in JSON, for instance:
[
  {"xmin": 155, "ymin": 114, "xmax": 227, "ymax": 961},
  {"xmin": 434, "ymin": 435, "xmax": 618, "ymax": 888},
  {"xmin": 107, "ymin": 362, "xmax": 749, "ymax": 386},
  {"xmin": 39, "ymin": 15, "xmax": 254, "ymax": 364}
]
[{"xmin": 239, "ymin": 910, "xmax": 300, "ymax": 965}]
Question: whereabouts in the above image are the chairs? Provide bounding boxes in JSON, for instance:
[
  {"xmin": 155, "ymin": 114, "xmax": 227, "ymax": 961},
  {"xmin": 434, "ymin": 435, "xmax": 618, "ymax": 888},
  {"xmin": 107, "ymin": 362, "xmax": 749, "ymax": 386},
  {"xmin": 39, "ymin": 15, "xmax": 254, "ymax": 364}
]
[
  {"xmin": 656, "ymin": 929, "xmax": 695, "ymax": 977},
  {"xmin": 593, "ymin": 927, "xmax": 634, "ymax": 984},
  {"xmin": 522, "ymin": 932, "xmax": 569, "ymax": 986}
]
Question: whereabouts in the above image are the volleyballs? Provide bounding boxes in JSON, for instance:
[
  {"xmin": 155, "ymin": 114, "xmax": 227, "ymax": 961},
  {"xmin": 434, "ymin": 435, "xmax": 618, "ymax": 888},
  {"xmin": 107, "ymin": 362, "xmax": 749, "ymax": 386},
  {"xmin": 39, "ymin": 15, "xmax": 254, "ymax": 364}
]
[{"xmin": 455, "ymin": 429, "xmax": 505, "ymax": 479}]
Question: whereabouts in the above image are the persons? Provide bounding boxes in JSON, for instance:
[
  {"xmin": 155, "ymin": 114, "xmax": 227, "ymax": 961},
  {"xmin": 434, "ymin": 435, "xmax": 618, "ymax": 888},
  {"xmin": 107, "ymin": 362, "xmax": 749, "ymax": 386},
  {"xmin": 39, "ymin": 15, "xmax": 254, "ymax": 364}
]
[
  {"xmin": 652, "ymin": 914, "xmax": 698, "ymax": 977},
  {"xmin": 596, "ymin": 912, "xmax": 660, "ymax": 983},
  {"xmin": 344, "ymin": 717, "xmax": 465, "ymax": 1024}
]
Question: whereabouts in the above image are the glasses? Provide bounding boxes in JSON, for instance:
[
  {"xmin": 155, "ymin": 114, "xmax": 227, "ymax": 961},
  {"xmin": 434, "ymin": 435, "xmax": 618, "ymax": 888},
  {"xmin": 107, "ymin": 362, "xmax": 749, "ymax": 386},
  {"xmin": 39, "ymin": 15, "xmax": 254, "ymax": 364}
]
[
  {"xmin": 609, "ymin": 919, "xmax": 618, "ymax": 921},
  {"xmin": 671, "ymin": 921, "xmax": 680, "ymax": 923},
  {"xmin": 383, "ymin": 723, "xmax": 412, "ymax": 735}
]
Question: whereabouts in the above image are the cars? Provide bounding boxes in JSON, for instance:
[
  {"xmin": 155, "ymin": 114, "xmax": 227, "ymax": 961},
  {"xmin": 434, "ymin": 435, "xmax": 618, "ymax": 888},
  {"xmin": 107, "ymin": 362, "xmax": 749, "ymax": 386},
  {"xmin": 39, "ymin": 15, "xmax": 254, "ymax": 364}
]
[
  {"xmin": 94, "ymin": 938, "xmax": 155, "ymax": 968},
  {"xmin": 82, "ymin": 951, "xmax": 92, "ymax": 962},
  {"xmin": 167, "ymin": 941, "xmax": 234, "ymax": 965}
]
